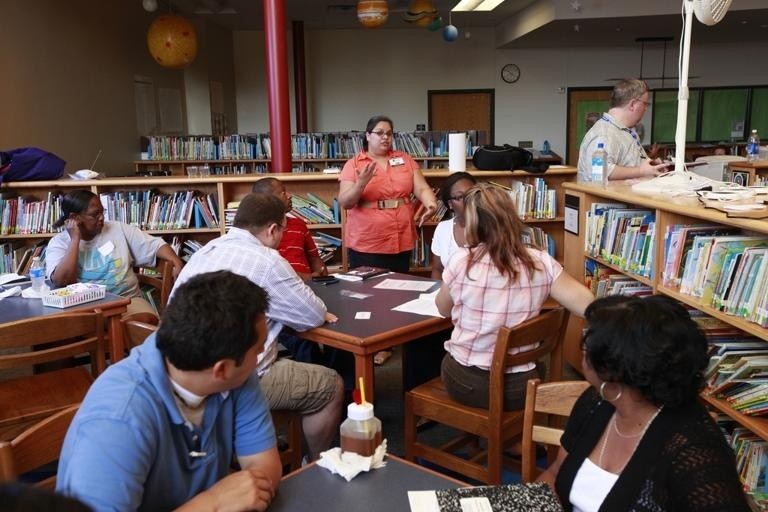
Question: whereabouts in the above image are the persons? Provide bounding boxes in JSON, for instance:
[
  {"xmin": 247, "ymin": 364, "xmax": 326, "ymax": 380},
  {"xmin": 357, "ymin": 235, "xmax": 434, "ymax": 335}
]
[
  {"xmin": 46, "ymin": 188, "xmax": 183, "ymax": 350},
  {"xmin": 714, "ymin": 145, "xmax": 732, "ymax": 157},
  {"xmin": 433, "ymin": 181, "xmax": 598, "ymax": 457},
  {"xmin": 577, "ymin": 77, "xmax": 671, "ymax": 183},
  {"xmin": 533, "ymin": 292, "xmax": 754, "ymax": 511},
  {"xmin": 333, "ymin": 116, "xmax": 439, "ymax": 273},
  {"xmin": 168, "ymin": 195, "xmax": 346, "ymax": 464},
  {"xmin": 249, "ymin": 177, "xmax": 354, "ymax": 425},
  {"xmin": 54, "ymin": 268, "xmax": 282, "ymax": 510}
]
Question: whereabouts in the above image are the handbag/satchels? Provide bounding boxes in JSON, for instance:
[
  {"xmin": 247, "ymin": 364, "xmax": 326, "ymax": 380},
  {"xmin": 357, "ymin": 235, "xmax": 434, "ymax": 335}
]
[
  {"xmin": 1, "ymin": 147, "xmax": 66, "ymax": 180},
  {"xmin": 473, "ymin": 144, "xmax": 533, "ymax": 171}
]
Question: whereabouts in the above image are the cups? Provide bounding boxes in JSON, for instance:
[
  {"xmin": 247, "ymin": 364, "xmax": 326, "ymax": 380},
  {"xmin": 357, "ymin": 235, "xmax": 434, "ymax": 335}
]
[{"xmin": 187, "ymin": 165, "xmax": 209, "ymax": 177}]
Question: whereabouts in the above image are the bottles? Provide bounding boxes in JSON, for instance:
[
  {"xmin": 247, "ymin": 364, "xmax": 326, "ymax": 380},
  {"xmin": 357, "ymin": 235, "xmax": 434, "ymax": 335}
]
[
  {"xmin": 29, "ymin": 256, "xmax": 45, "ymax": 291},
  {"xmin": 339, "ymin": 402, "xmax": 383, "ymax": 457},
  {"xmin": 591, "ymin": 144, "xmax": 609, "ymax": 187},
  {"xmin": 748, "ymin": 129, "xmax": 760, "ymax": 163}
]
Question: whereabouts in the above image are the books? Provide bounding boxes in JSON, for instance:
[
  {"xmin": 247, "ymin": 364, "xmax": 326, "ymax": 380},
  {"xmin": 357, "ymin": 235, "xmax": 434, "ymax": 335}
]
[
  {"xmin": 405, "ymin": 481, "xmax": 560, "ymax": 511},
  {"xmin": 584, "ymin": 172, "xmax": 767, "ymax": 506},
  {"xmin": 138, "ymin": 131, "xmax": 475, "ymax": 176},
  {"xmin": 1, "ymin": 177, "xmax": 559, "ymax": 274}
]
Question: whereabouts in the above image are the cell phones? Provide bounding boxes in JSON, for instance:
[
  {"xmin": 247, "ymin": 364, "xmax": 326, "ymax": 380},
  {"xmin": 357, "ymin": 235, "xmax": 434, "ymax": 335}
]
[{"xmin": 312, "ymin": 276, "xmax": 335, "ymax": 282}]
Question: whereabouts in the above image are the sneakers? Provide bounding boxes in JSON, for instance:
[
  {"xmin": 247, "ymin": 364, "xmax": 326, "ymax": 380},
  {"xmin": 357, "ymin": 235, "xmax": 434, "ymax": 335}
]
[
  {"xmin": 509, "ymin": 443, "xmax": 545, "ymax": 460},
  {"xmin": 465, "ymin": 434, "xmax": 487, "ymax": 451}
]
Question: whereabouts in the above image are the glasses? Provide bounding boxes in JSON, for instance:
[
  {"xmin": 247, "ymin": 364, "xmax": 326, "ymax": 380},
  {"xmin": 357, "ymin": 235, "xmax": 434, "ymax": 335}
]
[
  {"xmin": 451, "ymin": 196, "xmax": 465, "ymax": 201},
  {"xmin": 641, "ymin": 102, "xmax": 650, "ymax": 107},
  {"xmin": 83, "ymin": 208, "xmax": 106, "ymax": 216},
  {"xmin": 371, "ymin": 131, "xmax": 392, "ymax": 136}
]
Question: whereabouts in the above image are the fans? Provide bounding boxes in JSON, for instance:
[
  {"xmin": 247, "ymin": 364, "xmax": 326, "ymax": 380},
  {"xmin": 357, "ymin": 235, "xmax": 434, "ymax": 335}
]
[{"xmin": 633, "ymin": 0, "xmax": 734, "ymax": 194}]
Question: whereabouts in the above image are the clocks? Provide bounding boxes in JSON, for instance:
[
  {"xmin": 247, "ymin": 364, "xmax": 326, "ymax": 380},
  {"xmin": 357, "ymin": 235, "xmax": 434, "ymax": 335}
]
[{"xmin": 501, "ymin": 62, "xmax": 522, "ymax": 83}]
